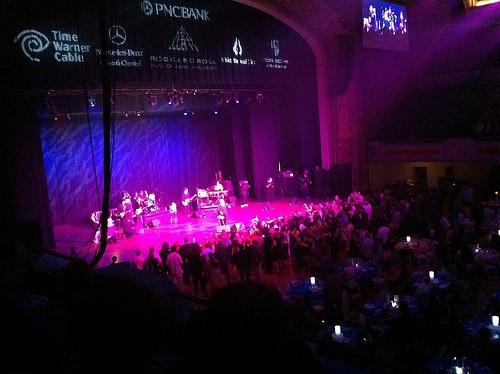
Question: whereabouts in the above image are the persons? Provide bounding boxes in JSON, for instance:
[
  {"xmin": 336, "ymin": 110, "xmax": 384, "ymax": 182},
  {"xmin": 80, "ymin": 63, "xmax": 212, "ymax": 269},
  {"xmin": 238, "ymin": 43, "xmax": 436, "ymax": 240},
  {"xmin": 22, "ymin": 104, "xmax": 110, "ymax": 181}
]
[
  {"xmin": 363, "ymin": 1, "xmax": 409, "ymax": 48},
  {"xmin": 10, "ymin": 125, "xmax": 499, "ymax": 374}
]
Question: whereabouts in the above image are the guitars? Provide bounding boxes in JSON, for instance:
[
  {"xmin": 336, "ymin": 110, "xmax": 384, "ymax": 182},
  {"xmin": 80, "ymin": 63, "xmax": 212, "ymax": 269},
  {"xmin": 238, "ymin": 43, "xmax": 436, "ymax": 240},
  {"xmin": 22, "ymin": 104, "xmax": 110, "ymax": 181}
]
[
  {"xmin": 182, "ymin": 194, "xmax": 197, "ymax": 206},
  {"xmin": 115, "ymin": 210, "xmax": 130, "ymax": 218},
  {"xmin": 135, "ymin": 205, "xmax": 148, "ymax": 215}
]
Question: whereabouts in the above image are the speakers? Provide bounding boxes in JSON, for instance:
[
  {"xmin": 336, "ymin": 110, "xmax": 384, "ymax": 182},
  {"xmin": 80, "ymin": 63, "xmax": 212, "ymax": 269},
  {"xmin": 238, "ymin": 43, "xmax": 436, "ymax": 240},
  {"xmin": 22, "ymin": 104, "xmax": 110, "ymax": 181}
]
[{"xmin": 330, "ymin": 162, "xmax": 353, "ymax": 198}]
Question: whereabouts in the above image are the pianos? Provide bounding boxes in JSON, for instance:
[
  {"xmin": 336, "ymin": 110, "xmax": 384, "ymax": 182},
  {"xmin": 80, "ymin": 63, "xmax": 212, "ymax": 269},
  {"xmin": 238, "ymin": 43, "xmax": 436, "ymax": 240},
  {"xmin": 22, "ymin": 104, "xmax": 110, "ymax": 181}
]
[{"xmin": 207, "ymin": 190, "xmax": 228, "ymax": 194}]
